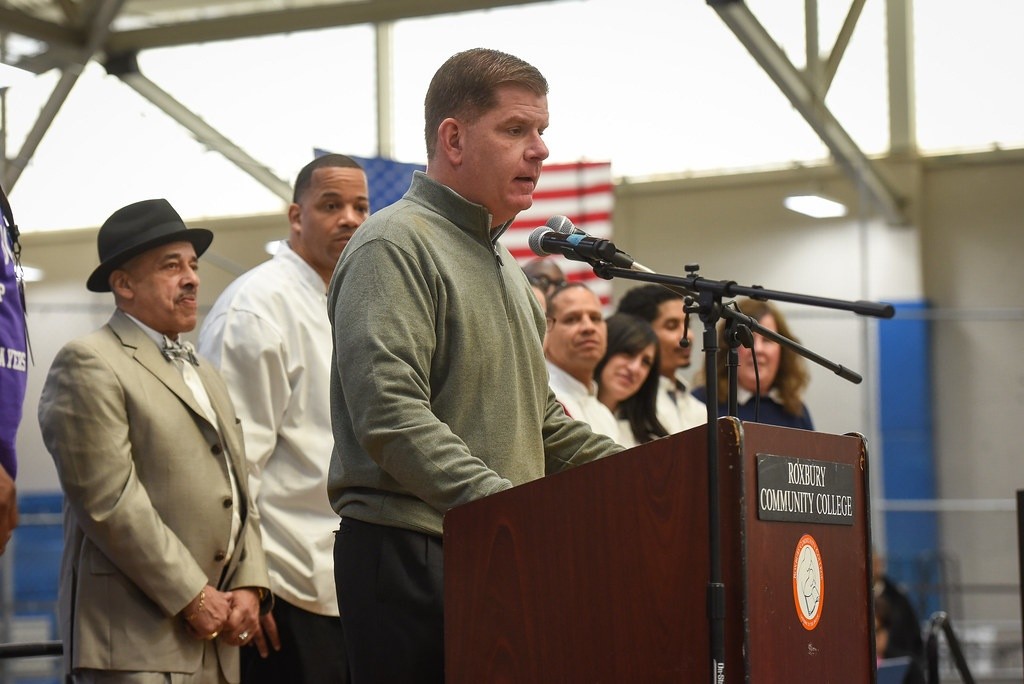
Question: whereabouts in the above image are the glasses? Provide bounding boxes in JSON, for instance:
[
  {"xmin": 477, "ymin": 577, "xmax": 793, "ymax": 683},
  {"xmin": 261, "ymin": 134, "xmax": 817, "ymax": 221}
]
[{"xmin": 526, "ymin": 272, "xmax": 566, "ymax": 294}]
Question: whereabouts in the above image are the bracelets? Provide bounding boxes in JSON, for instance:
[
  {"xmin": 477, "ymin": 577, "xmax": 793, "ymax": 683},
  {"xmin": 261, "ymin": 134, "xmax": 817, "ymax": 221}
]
[{"xmin": 183, "ymin": 586, "xmax": 205, "ymax": 619}]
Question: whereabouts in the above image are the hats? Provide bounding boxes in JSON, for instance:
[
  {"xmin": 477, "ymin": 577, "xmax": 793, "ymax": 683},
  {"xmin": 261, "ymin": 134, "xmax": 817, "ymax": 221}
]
[{"xmin": 86, "ymin": 198, "xmax": 212, "ymax": 292}]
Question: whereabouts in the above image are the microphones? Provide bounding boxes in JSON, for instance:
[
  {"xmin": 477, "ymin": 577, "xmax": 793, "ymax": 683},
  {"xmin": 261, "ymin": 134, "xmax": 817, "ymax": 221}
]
[{"xmin": 528, "ymin": 214, "xmax": 635, "ymax": 269}]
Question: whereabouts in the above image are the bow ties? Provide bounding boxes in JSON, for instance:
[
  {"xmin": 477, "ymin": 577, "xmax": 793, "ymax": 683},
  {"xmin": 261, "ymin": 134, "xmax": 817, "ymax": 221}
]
[{"xmin": 161, "ymin": 334, "xmax": 200, "ymax": 367}]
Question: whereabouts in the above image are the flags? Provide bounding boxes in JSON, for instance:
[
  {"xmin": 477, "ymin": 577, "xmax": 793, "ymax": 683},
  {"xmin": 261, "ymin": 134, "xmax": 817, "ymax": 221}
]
[{"xmin": 312, "ymin": 148, "xmax": 614, "ymax": 320}]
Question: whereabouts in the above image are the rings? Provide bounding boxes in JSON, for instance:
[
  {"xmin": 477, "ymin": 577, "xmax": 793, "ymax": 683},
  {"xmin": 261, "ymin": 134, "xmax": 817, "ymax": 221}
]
[
  {"xmin": 237, "ymin": 631, "xmax": 248, "ymax": 640},
  {"xmin": 206, "ymin": 632, "xmax": 217, "ymax": 640}
]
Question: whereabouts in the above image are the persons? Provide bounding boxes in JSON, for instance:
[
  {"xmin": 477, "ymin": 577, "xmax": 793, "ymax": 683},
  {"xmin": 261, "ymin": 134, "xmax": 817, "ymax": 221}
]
[
  {"xmin": 0, "ymin": 188, "xmax": 34, "ymax": 554},
  {"xmin": 689, "ymin": 297, "xmax": 815, "ymax": 431},
  {"xmin": 37, "ymin": 199, "xmax": 274, "ymax": 684},
  {"xmin": 873, "ymin": 549, "xmax": 921, "ymax": 658},
  {"xmin": 327, "ymin": 47, "xmax": 630, "ymax": 684},
  {"xmin": 618, "ymin": 284, "xmax": 709, "ymax": 432},
  {"xmin": 520, "ymin": 257, "xmax": 671, "ymax": 452},
  {"xmin": 199, "ymin": 155, "xmax": 371, "ymax": 684}
]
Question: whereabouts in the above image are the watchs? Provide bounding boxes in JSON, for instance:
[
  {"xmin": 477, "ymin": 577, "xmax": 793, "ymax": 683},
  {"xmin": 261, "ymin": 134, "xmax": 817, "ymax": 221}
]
[{"xmin": 258, "ymin": 587, "xmax": 268, "ymax": 603}]
